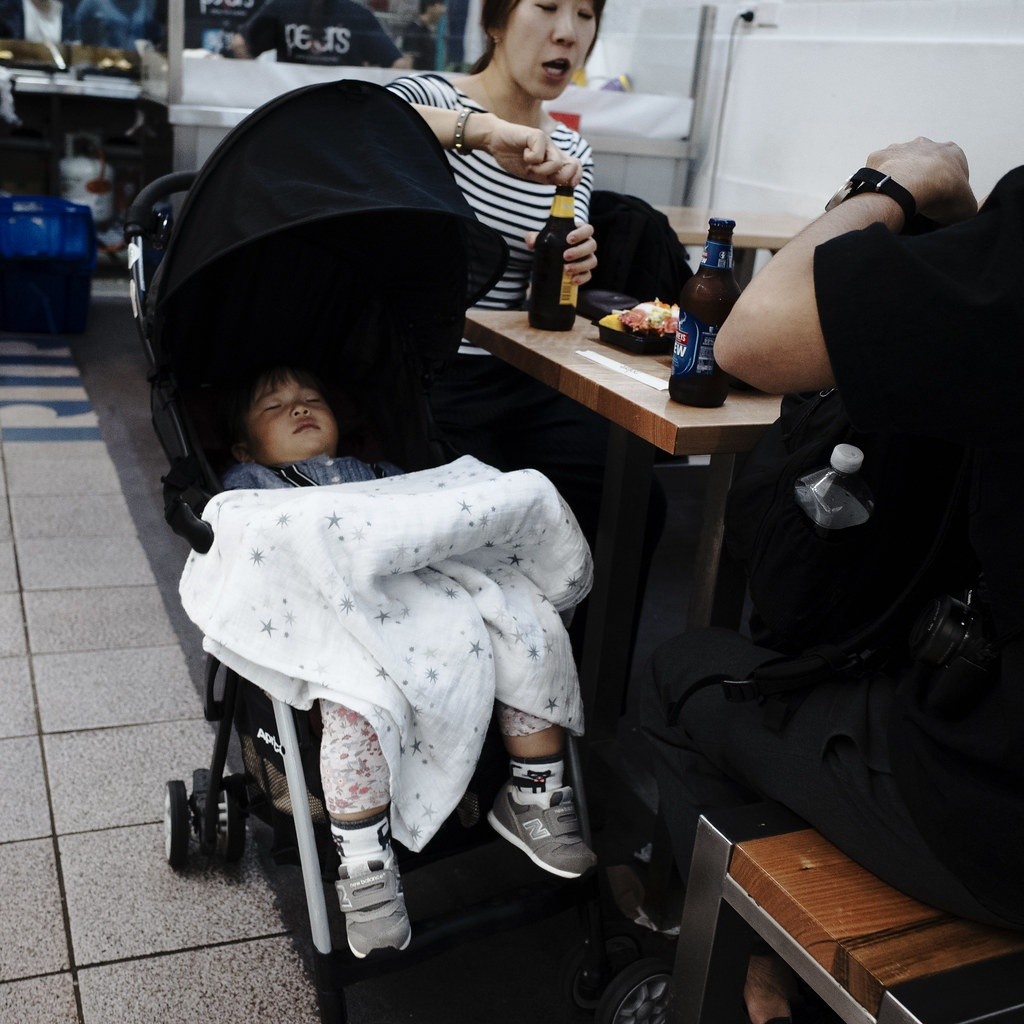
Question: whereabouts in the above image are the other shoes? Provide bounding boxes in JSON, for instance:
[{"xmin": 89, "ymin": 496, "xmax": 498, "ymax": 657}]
[{"xmin": 738, "ymin": 990, "xmax": 794, "ymax": 1024}]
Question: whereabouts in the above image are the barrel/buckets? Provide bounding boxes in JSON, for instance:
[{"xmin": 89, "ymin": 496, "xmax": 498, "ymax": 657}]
[{"xmin": 60, "ymin": 133, "xmax": 114, "ymax": 221}]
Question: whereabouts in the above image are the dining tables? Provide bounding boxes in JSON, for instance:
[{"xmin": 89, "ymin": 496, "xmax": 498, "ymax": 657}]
[
  {"xmin": 469, "ymin": 302, "xmax": 795, "ymax": 803},
  {"xmin": 657, "ymin": 207, "xmax": 806, "ymax": 246}
]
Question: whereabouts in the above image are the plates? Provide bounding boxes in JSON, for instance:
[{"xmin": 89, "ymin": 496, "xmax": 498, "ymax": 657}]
[{"xmin": 590, "ymin": 318, "xmax": 677, "ymax": 355}]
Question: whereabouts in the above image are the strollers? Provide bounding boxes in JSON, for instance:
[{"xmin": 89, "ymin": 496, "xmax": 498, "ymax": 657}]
[{"xmin": 126, "ymin": 77, "xmax": 676, "ymax": 1024}]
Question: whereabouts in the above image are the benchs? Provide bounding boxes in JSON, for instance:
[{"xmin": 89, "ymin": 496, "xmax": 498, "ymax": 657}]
[{"xmin": 665, "ymin": 804, "xmax": 1024, "ymax": 1023}]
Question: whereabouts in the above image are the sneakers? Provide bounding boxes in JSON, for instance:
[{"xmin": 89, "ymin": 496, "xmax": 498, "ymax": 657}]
[
  {"xmin": 335, "ymin": 844, "xmax": 413, "ymax": 957},
  {"xmin": 487, "ymin": 778, "xmax": 600, "ymax": 879}
]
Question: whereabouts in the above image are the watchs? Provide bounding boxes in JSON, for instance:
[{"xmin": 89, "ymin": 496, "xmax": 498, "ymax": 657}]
[{"xmin": 824, "ymin": 166, "xmax": 918, "ymax": 234}]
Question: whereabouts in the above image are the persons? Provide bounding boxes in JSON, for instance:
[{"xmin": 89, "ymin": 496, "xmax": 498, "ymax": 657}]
[
  {"xmin": 0, "ymin": 0, "xmax": 168, "ymax": 194},
  {"xmin": 224, "ymin": 0, "xmax": 413, "ymax": 70},
  {"xmin": 401, "ymin": 1, "xmax": 447, "ymax": 72},
  {"xmin": 379, "ymin": 0, "xmax": 606, "ymax": 616},
  {"xmin": 640, "ymin": 140, "xmax": 1023, "ymax": 1024},
  {"xmin": 223, "ymin": 350, "xmax": 600, "ymax": 964}
]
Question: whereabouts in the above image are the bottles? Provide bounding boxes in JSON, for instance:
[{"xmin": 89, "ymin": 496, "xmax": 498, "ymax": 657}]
[
  {"xmin": 528, "ymin": 179, "xmax": 581, "ymax": 330},
  {"xmin": 669, "ymin": 217, "xmax": 741, "ymax": 406}
]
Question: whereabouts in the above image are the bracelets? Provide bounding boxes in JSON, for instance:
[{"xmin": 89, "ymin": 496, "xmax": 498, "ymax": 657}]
[{"xmin": 453, "ymin": 109, "xmax": 473, "ymax": 154}]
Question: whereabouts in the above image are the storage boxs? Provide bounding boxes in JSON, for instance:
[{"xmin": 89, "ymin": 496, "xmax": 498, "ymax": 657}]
[{"xmin": 0, "ymin": 194, "xmax": 95, "ymax": 333}]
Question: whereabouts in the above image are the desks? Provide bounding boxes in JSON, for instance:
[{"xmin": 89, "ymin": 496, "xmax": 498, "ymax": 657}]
[{"xmin": 0, "ymin": 67, "xmax": 141, "ymax": 197}]
